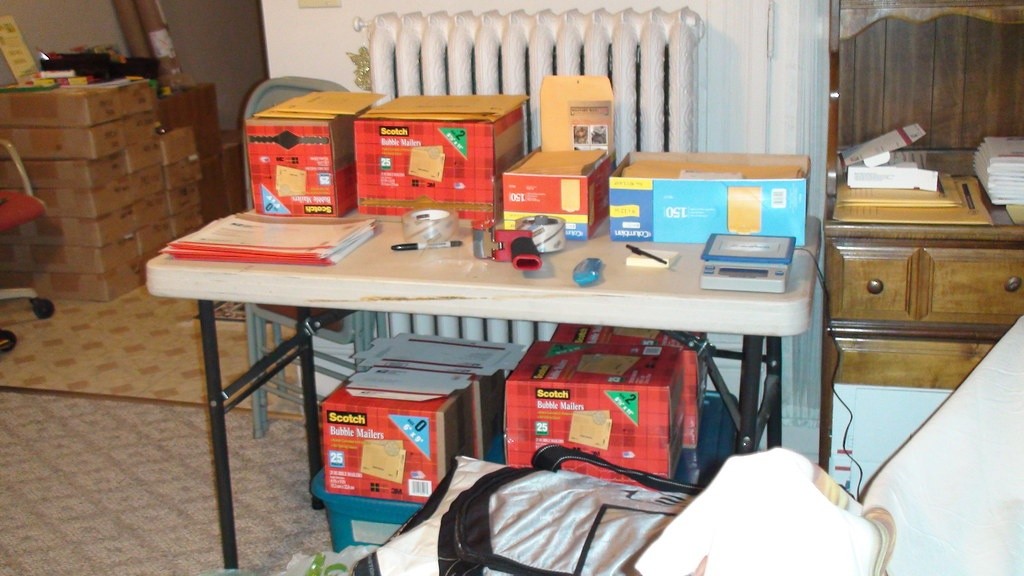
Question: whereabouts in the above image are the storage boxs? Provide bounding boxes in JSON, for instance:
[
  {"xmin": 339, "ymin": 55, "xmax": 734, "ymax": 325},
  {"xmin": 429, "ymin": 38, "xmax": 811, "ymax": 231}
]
[
  {"xmin": 320, "ymin": 370, "xmax": 474, "ymax": 505},
  {"xmin": 538, "ymin": 75, "xmax": 615, "ymax": 155},
  {"xmin": 503, "ymin": 340, "xmax": 685, "ymax": 492},
  {"xmin": 245, "ymin": 94, "xmax": 372, "ymax": 218},
  {"xmin": 549, "ymin": 323, "xmax": 709, "ymax": 450},
  {"xmin": 0, "ymin": 74, "xmax": 246, "ymax": 304},
  {"xmin": 350, "ymin": 332, "xmax": 506, "ymax": 460},
  {"xmin": 353, "ymin": 95, "xmax": 527, "ymax": 230},
  {"xmin": 607, "ymin": 151, "xmax": 811, "ymax": 249},
  {"xmin": 311, "ymin": 466, "xmax": 423, "ymax": 553},
  {"xmin": 502, "ymin": 149, "xmax": 617, "ymax": 242}
]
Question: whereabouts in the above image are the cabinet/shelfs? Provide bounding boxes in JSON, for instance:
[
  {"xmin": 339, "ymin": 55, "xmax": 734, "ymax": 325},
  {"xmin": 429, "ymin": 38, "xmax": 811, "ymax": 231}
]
[{"xmin": 822, "ymin": 222, "xmax": 1024, "ymax": 391}]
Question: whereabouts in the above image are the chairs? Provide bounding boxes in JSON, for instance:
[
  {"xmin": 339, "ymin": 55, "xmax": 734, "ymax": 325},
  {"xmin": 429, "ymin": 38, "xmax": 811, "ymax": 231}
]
[
  {"xmin": 241, "ymin": 77, "xmax": 387, "ymax": 438},
  {"xmin": 351, "ymin": 312, "xmax": 1023, "ymax": 574}
]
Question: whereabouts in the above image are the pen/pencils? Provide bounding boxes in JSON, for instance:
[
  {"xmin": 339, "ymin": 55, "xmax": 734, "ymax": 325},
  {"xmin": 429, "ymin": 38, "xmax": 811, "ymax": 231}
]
[
  {"xmin": 937, "ymin": 177, "xmax": 946, "ymax": 199},
  {"xmin": 626, "ymin": 244, "xmax": 668, "ymax": 264}
]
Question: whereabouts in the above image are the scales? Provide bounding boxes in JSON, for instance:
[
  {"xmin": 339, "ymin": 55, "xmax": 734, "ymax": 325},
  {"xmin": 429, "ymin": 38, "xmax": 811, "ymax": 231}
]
[{"xmin": 699, "ymin": 234, "xmax": 796, "ymax": 293}]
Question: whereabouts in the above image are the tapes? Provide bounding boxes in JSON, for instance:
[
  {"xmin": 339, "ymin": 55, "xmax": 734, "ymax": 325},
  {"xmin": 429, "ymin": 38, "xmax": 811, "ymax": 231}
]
[
  {"xmin": 518, "ymin": 214, "xmax": 567, "ymax": 254},
  {"xmin": 401, "ymin": 206, "xmax": 458, "ymax": 242}
]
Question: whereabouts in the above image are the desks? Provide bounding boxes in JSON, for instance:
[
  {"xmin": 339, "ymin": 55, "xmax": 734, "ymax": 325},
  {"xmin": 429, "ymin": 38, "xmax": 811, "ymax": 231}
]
[{"xmin": 144, "ymin": 206, "xmax": 826, "ymax": 572}]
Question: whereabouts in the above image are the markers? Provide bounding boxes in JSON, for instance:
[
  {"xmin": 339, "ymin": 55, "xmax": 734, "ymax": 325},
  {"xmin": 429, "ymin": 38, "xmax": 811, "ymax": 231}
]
[{"xmin": 391, "ymin": 240, "xmax": 463, "ymax": 251}]
